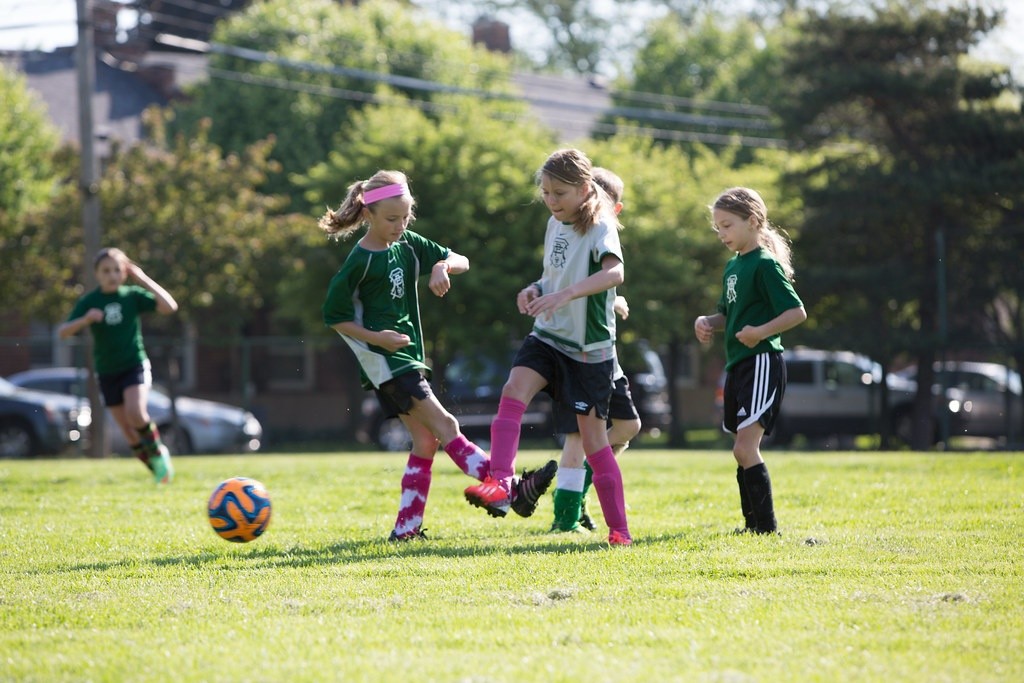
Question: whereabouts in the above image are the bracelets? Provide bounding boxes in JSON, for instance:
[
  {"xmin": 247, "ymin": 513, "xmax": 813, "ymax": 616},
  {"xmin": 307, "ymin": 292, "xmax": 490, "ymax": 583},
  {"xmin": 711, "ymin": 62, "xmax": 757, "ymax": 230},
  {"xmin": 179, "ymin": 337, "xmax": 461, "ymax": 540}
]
[{"xmin": 438, "ymin": 261, "xmax": 451, "ymax": 272}]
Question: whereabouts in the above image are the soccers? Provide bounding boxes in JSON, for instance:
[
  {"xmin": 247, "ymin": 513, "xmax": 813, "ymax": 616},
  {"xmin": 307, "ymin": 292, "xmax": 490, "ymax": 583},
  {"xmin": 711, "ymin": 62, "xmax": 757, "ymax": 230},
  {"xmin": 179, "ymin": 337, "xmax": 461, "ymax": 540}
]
[{"xmin": 206, "ymin": 475, "xmax": 273, "ymax": 544}]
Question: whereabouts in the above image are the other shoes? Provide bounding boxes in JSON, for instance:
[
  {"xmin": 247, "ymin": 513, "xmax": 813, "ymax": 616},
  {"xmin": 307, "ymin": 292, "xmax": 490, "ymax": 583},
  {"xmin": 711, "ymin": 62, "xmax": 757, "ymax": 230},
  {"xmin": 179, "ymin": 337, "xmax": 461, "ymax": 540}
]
[{"xmin": 149, "ymin": 444, "xmax": 172, "ymax": 484}]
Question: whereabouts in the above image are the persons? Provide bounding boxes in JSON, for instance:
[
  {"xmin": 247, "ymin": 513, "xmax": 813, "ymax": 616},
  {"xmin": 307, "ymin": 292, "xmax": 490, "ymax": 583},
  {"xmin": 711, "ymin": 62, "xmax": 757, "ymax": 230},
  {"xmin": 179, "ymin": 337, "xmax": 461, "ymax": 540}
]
[
  {"xmin": 58, "ymin": 247, "xmax": 178, "ymax": 485},
  {"xmin": 464, "ymin": 150, "xmax": 634, "ymax": 546},
  {"xmin": 323, "ymin": 170, "xmax": 557, "ymax": 543},
  {"xmin": 695, "ymin": 188, "xmax": 807, "ymax": 536},
  {"xmin": 553, "ymin": 168, "xmax": 641, "ymax": 533}
]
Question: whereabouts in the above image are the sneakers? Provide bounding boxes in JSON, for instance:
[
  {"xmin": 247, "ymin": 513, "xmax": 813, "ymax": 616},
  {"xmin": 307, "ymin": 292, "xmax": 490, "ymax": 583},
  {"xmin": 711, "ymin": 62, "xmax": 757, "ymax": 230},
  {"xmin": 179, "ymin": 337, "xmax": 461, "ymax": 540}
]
[
  {"xmin": 389, "ymin": 528, "xmax": 428, "ymax": 542},
  {"xmin": 465, "ymin": 471, "xmax": 510, "ymax": 518},
  {"xmin": 510, "ymin": 459, "xmax": 558, "ymax": 518},
  {"xmin": 578, "ymin": 506, "xmax": 597, "ymax": 530},
  {"xmin": 549, "ymin": 523, "xmax": 590, "ymax": 535},
  {"xmin": 602, "ymin": 531, "xmax": 632, "ymax": 545}
]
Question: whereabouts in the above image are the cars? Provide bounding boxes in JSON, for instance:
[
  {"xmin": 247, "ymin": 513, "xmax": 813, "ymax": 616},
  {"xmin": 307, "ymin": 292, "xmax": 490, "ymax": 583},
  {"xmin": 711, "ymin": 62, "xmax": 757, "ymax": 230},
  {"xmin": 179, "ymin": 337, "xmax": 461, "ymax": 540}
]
[
  {"xmin": 890, "ymin": 361, "xmax": 1022, "ymax": 449},
  {"xmin": 6, "ymin": 364, "xmax": 268, "ymax": 456},
  {"xmin": 0, "ymin": 380, "xmax": 92, "ymax": 461}
]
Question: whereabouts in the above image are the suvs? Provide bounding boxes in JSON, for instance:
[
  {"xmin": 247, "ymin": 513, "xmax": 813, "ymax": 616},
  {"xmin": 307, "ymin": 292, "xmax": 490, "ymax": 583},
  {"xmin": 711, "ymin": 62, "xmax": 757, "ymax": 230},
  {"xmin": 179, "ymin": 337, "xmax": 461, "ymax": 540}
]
[
  {"xmin": 353, "ymin": 328, "xmax": 673, "ymax": 452},
  {"xmin": 714, "ymin": 345, "xmax": 969, "ymax": 449}
]
